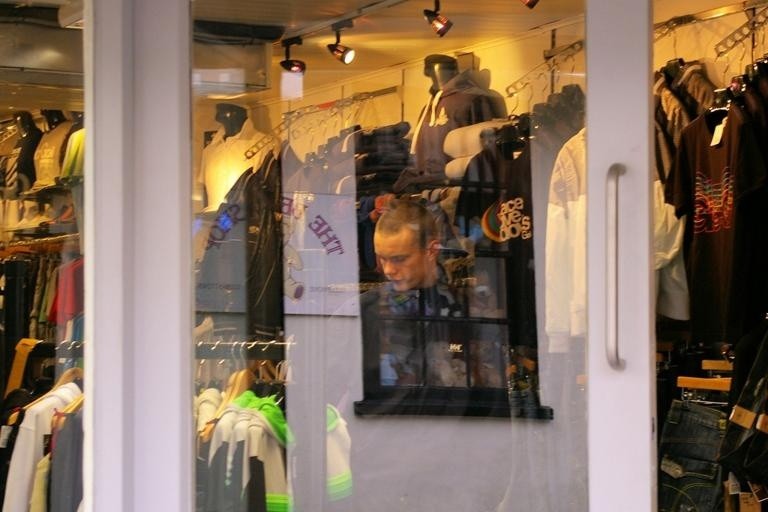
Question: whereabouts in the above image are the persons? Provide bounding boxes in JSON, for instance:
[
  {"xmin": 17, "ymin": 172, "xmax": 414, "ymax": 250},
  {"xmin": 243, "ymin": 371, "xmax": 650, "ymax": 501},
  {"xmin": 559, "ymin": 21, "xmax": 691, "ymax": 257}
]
[
  {"xmin": 329, "ymin": 201, "xmax": 502, "ymax": 387},
  {"xmin": 11, "ymin": 113, "xmax": 88, "ymax": 185},
  {"xmin": 415, "ymin": 54, "xmax": 488, "ymax": 168},
  {"xmin": 197, "ymin": 106, "xmax": 277, "ymax": 196}
]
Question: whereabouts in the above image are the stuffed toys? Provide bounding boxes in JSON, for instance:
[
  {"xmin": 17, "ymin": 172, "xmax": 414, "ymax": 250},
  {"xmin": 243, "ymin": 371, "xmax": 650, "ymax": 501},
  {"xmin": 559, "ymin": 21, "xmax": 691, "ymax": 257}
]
[{"xmin": 380, "ymin": 270, "xmax": 505, "ymax": 388}]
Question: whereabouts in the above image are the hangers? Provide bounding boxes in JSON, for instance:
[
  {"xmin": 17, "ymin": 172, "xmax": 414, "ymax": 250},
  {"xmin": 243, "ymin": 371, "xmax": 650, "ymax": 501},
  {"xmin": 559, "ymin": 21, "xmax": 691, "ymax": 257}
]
[
  {"xmin": 241, "ymin": 97, "xmax": 367, "ymax": 179},
  {"xmin": 8, "ymin": 337, "xmax": 297, "ymax": 445},
  {"xmin": 493, "ymin": 20, "xmax": 768, "ymax": 146}
]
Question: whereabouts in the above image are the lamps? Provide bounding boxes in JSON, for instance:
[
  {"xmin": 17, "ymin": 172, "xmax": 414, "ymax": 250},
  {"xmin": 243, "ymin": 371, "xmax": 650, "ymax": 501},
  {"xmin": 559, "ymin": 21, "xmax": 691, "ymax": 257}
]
[{"xmin": 272, "ymin": 0, "xmax": 539, "ymax": 72}]
